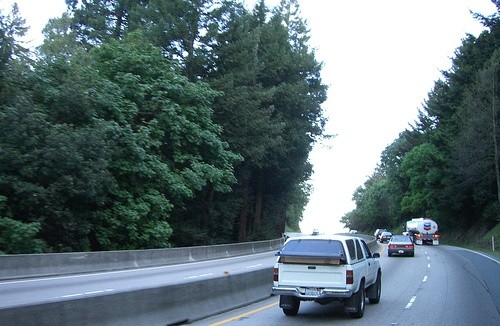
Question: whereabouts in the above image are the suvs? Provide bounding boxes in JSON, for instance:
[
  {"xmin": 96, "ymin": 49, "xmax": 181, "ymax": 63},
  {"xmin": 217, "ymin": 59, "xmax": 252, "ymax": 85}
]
[
  {"xmin": 270, "ymin": 235, "xmax": 382, "ymax": 319},
  {"xmin": 374, "ymin": 229, "xmax": 387, "ymax": 241}
]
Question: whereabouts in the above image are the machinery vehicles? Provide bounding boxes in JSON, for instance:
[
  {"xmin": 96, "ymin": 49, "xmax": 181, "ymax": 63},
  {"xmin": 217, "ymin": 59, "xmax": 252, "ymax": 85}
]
[{"xmin": 402, "ymin": 218, "xmax": 441, "ymax": 245}]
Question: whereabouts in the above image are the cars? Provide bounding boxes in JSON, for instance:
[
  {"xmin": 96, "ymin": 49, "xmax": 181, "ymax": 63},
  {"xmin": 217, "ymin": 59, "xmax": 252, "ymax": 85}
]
[
  {"xmin": 380, "ymin": 231, "xmax": 393, "ymax": 243},
  {"xmin": 350, "ymin": 230, "xmax": 357, "ymax": 234},
  {"xmin": 285, "ymin": 233, "xmax": 301, "ymax": 241},
  {"xmin": 387, "ymin": 235, "xmax": 415, "ymax": 257}
]
